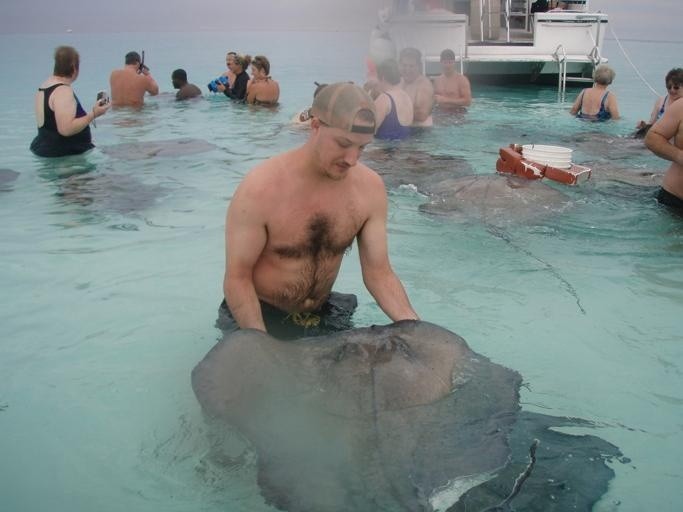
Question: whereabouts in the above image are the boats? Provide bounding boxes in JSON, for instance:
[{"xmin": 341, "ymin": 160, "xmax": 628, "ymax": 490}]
[{"xmin": 378, "ymin": 0, "xmax": 612, "ymax": 92}]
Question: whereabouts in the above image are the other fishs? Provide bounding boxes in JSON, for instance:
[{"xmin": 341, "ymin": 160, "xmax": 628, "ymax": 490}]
[
  {"xmin": 190, "ymin": 316, "xmax": 632, "ymax": 512},
  {"xmin": 364, "ymin": 129, "xmax": 659, "ymax": 230},
  {"xmin": 57, "ymin": 137, "xmax": 217, "ymax": 219}
]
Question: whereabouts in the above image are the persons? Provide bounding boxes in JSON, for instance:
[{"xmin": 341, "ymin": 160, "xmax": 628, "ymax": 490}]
[
  {"xmin": 431, "ymin": 49, "xmax": 472, "ymax": 109},
  {"xmin": 289, "ymin": 83, "xmax": 327, "ymax": 125},
  {"xmin": 171, "ymin": 68, "xmax": 201, "ymax": 101},
  {"xmin": 367, "ymin": 58, "xmax": 411, "ymax": 138},
  {"xmin": 365, "ymin": 81, "xmax": 380, "ymax": 98},
  {"xmin": 634, "ymin": 68, "xmax": 683, "ymax": 140},
  {"xmin": 643, "ymin": 95, "xmax": 683, "ymax": 217},
  {"xmin": 214, "ymin": 84, "xmax": 419, "ymax": 340},
  {"xmin": 108, "ymin": 51, "xmax": 158, "ymax": 109},
  {"xmin": 245, "ymin": 56, "xmax": 279, "ymax": 105},
  {"xmin": 28, "ymin": 46, "xmax": 112, "ymax": 158},
  {"xmin": 398, "ymin": 48, "xmax": 434, "ymax": 127},
  {"xmin": 215, "ymin": 55, "xmax": 250, "ymax": 101},
  {"xmin": 569, "ymin": 64, "xmax": 621, "ymax": 122},
  {"xmin": 219, "ymin": 51, "xmax": 236, "ymax": 89}
]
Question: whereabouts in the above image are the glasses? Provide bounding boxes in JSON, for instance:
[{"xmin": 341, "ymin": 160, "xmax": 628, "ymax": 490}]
[{"xmin": 666, "ymin": 84, "xmax": 679, "ymax": 90}]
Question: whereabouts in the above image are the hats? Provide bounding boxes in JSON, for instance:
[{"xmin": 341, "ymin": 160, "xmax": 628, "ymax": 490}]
[{"xmin": 300, "ymin": 80, "xmax": 376, "ymax": 134}]
